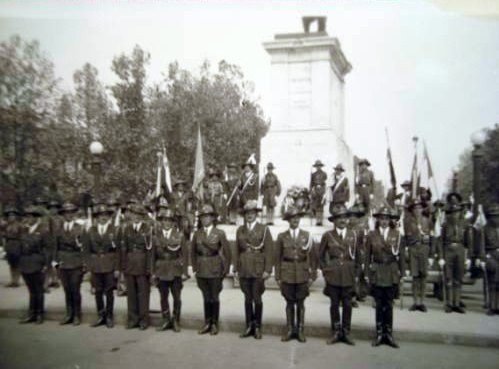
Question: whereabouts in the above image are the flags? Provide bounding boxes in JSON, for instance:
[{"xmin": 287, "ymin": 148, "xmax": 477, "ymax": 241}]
[
  {"xmin": 155, "ymin": 128, "xmax": 204, "ymax": 195},
  {"xmin": 384, "ymin": 139, "xmax": 434, "ymax": 200}
]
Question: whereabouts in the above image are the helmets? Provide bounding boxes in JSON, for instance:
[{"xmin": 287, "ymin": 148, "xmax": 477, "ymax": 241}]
[{"xmin": 4, "ymin": 159, "xmax": 499, "ymax": 218}]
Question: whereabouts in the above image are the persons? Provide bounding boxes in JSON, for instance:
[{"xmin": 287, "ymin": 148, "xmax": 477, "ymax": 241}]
[
  {"xmin": 52, "ymin": 203, "xmax": 87, "ymax": 325},
  {"xmin": 310, "ymin": 159, "xmax": 327, "ymax": 226},
  {"xmin": 329, "ymin": 164, "xmax": 350, "ymax": 212},
  {"xmin": 85, "ymin": 204, "xmax": 122, "ymax": 329},
  {"xmin": 353, "ymin": 159, "xmax": 374, "ymax": 207},
  {"xmin": 364, "ymin": 206, "xmax": 404, "ymax": 350},
  {"xmin": 18, "ymin": 206, "xmax": 50, "ymax": 324},
  {"xmin": 120, "ymin": 204, "xmax": 153, "ymax": 330},
  {"xmin": 3, "ymin": 206, "xmax": 21, "ymax": 287},
  {"xmin": 318, "ymin": 203, "xmax": 361, "ymax": 346},
  {"xmin": 274, "ymin": 207, "xmax": 316, "ymax": 342},
  {"xmin": 191, "ymin": 206, "xmax": 230, "ymax": 334},
  {"xmin": 260, "ymin": 161, "xmax": 281, "ymax": 226},
  {"xmin": 36, "ymin": 162, "xmax": 240, "ymax": 296},
  {"xmin": 153, "ymin": 210, "xmax": 188, "ymax": 332},
  {"xmin": 344, "ymin": 181, "xmax": 499, "ymax": 317},
  {"xmin": 239, "ymin": 162, "xmax": 259, "ymax": 215},
  {"xmin": 233, "ymin": 201, "xmax": 273, "ymax": 339}
]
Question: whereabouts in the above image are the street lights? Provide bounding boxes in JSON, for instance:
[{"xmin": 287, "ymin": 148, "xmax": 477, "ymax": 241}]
[
  {"xmin": 90, "ymin": 141, "xmax": 103, "ymax": 203},
  {"xmin": 471, "ymin": 131, "xmax": 484, "ymax": 277}
]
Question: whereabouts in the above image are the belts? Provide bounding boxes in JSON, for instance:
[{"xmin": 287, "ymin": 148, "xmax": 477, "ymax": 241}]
[
  {"xmin": 283, "ymin": 256, "xmax": 308, "ymax": 264},
  {"xmin": 90, "ymin": 248, "xmax": 118, "ymax": 255},
  {"xmin": 156, "ymin": 254, "xmax": 180, "ymax": 260},
  {"xmin": 126, "ymin": 247, "xmax": 148, "ymax": 254},
  {"xmin": 372, "ymin": 256, "xmax": 398, "ymax": 264},
  {"xmin": 239, "ymin": 247, "xmax": 263, "ymax": 253},
  {"xmin": 58, "ymin": 246, "xmax": 82, "ymax": 253},
  {"xmin": 197, "ymin": 250, "xmax": 219, "ymax": 257},
  {"xmin": 328, "ymin": 254, "xmax": 353, "ymax": 260},
  {"xmin": 22, "ymin": 250, "xmax": 41, "ymax": 256}
]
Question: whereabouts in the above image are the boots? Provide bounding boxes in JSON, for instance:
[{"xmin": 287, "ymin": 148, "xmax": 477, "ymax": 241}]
[
  {"xmin": 19, "ymin": 295, "xmax": 44, "ymax": 325},
  {"xmin": 370, "ymin": 312, "xmax": 398, "ymax": 348},
  {"xmin": 238, "ymin": 302, "xmax": 264, "ymax": 340},
  {"xmin": 326, "ymin": 306, "xmax": 356, "ymax": 345},
  {"xmin": 90, "ymin": 296, "xmax": 116, "ymax": 328},
  {"xmin": 199, "ymin": 300, "xmax": 220, "ymax": 335},
  {"xmin": 280, "ymin": 306, "xmax": 305, "ymax": 343},
  {"xmin": 156, "ymin": 299, "xmax": 182, "ymax": 332},
  {"xmin": 60, "ymin": 295, "xmax": 82, "ymax": 326}
]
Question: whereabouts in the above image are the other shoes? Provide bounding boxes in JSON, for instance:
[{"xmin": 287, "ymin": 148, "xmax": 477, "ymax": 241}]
[
  {"xmin": 482, "ymin": 303, "xmax": 499, "ymax": 316},
  {"xmin": 408, "ymin": 303, "xmax": 427, "ymax": 312},
  {"xmin": 125, "ymin": 324, "xmax": 148, "ymax": 331},
  {"xmin": 434, "ymin": 293, "xmax": 467, "ymax": 314}
]
